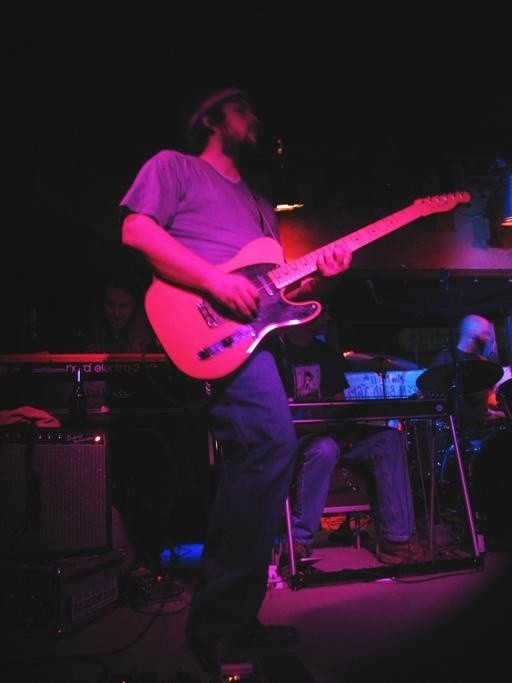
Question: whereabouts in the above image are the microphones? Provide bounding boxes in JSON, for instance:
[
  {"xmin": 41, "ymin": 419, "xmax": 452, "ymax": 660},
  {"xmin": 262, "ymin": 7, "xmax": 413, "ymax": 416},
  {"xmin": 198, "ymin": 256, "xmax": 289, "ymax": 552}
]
[
  {"xmin": 342, "ymin": 350, "xmax": 376, "ymax": 362},
  {"xmin": 439, "ymin": 267, "xmax": 452, "ymax": 295}
]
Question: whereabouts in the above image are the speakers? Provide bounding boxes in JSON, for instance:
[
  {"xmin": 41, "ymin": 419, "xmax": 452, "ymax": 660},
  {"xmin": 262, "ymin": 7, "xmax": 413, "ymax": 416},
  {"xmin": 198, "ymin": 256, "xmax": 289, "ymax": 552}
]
[
  {"xmin": 1, "ymin": 426, "xmax": 112, "ymax": 565},
  {"xmin": 47, "ymin": 410, "xmax": 209, "ymax": 552}
]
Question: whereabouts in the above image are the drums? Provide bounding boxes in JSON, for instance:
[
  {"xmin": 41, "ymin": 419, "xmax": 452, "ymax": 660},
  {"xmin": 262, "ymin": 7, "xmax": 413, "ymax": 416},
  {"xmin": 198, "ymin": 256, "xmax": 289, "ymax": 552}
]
[
  {"xmin": 496, "ymin": 378, "xmax": 512, "ymax": 417},
  {"xmin": 438, "ymin": 418, "xmax": 512, "ymax": 554}
]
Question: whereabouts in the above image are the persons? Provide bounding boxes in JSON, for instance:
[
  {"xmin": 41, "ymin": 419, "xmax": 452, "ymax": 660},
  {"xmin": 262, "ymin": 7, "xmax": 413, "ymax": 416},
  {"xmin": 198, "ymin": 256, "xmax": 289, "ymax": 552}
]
[
  {"xmin": 106, "ymin": 74, "xmax": 358, "ymax": 681},
  {"xmin": 73, "ymin": 271, "xmax": 156, "ymax": 359},
  {"xmin": 262, "ymin": 269, "xmax": 438, "ymax": 588},
  {"xmin": 422, "ymin": 308, "xmax": 511, "ymax": 421}
]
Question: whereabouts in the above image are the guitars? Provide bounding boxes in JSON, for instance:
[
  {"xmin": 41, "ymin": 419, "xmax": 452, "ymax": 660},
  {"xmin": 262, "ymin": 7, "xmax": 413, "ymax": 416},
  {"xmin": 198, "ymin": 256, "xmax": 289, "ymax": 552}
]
[{"xmin": 144, "ymin": 192, "xmax": 471, "ymax": 383}]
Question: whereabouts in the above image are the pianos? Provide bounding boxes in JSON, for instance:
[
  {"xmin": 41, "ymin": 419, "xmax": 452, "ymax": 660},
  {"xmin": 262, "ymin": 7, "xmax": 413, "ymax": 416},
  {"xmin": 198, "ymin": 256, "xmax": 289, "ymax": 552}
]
[
  {"xmin": 288, "ymin": 399, "xmax": 453, "ymax": 423},
  {"xmin": 0, "ymin": 354, "xmax": 173, "ymax": 380}
]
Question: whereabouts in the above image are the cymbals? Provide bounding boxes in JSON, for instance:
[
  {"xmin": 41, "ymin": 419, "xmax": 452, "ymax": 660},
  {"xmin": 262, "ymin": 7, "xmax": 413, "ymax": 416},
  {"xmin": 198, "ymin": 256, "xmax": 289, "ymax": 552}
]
[
  {"xmin": 415, "ymin": 360, "xmax": 503, "ymax": 394},
  {"xmin": 347, "ymin": 354, "xmax": 419, "ymax": 369}
]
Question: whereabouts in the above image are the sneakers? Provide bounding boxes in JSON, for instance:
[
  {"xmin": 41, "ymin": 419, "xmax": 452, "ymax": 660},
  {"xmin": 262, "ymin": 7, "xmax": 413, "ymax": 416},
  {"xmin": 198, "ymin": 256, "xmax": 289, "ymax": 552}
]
[
  {"xmin": 379, "ymin": 540, "xmax": 440, "ymax": 563},
  {"xmin": 280, "ymin": 543, "xmax": 307, "ymax": 565},
  {"xmin": 204, "ymin": 639, "xmax": 265, "ymax": 681},
  {"xmin": 247, "ymin": 620, "xmax": 299, "ymax": 657}
]
